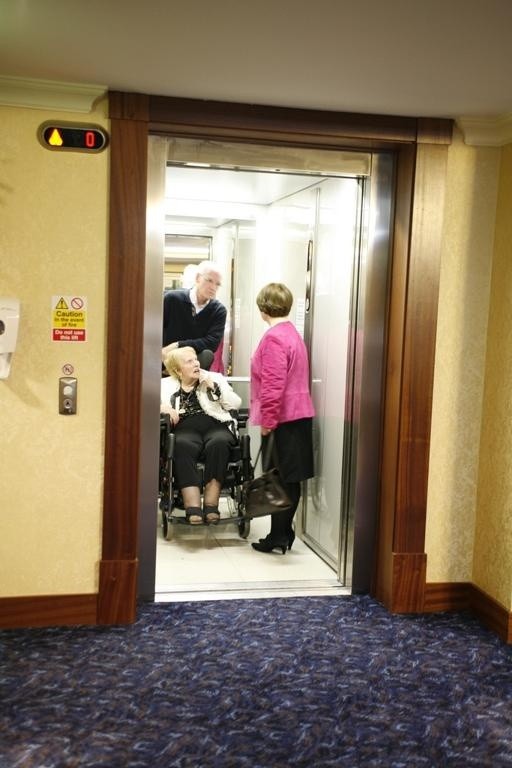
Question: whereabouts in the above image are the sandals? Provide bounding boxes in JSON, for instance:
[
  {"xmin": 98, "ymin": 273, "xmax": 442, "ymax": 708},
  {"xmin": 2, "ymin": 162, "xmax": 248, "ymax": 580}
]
[
  {"xmin": 204, "ymin": 504, "xmax": 220, "ymax": 524},
  {"xmin": 186, "ymin": 506, "xmax": 204, "ymax": 525}
]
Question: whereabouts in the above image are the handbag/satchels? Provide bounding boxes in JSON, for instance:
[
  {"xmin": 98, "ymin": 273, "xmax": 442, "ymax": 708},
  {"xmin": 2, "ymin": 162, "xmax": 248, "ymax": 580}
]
[{"xmin": 242, "ymin": 468, "xmax": 293, "ymax": 516}]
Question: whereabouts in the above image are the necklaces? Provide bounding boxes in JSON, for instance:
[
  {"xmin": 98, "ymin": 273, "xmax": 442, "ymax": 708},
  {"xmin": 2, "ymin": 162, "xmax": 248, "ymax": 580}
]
[{"xmin": 178, "ymin": 383, "xmax": 197, "ymax": 414}]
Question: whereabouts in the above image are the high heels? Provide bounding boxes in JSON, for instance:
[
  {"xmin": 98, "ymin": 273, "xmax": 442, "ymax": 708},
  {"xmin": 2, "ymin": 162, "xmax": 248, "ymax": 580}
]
[
  {"xmin": 259, "ymin": 531, "xmax": 294, "ymax": 549},
  {"xmin": 251, "ymin": 539, "xmax": 287, "ymax": 555}
]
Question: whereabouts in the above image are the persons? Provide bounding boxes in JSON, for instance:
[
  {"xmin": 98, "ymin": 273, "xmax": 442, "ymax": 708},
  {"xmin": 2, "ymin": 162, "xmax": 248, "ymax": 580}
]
[
  {"xmin": 160, "ymin": 345, "xmax": 243, "ymax": 526},
  {"xmin": 162, "ymin": 263, "xmax": 228, "ymax": 379},
  {"xmin": 181, "ymin": 263, "xmax": 226, "ymax": 376},
  {"xmin": 246, "ymin": 281, "xmax": 317, "ymax": 555}
]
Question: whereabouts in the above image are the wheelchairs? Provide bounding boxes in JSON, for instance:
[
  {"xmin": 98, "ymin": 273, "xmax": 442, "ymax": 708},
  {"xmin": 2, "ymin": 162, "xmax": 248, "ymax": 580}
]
[{"xmin": 160, "ymin": 405, "xmax": 254, "ymax": 539}]
